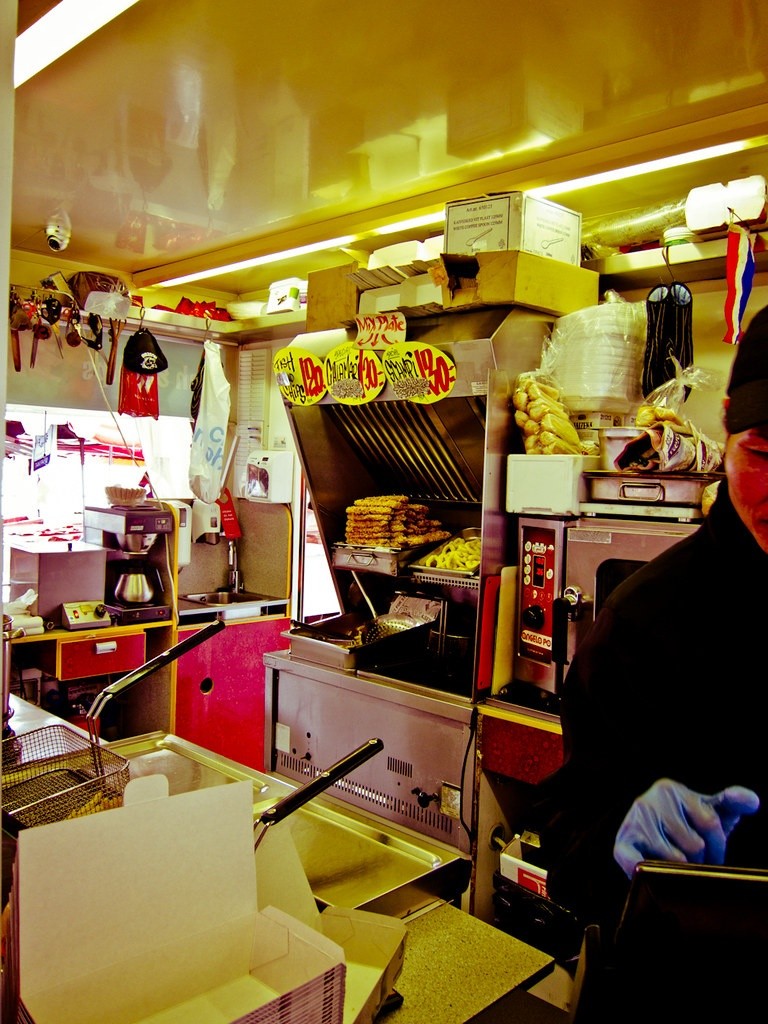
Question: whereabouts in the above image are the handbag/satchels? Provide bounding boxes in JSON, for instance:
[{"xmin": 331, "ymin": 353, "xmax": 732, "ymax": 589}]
[
  {"xmin": 118, "ymin": 363, "xmax": 159, "ymax": 420},
  {"xmin": 125, "ymin": 328, "xmax": 168, "ymax": 374}
]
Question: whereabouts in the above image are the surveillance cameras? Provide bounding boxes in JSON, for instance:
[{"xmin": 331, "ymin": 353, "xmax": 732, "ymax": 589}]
[{"xmin": 45, "ymin": 226, "xmax": 72, "ymax": 252}]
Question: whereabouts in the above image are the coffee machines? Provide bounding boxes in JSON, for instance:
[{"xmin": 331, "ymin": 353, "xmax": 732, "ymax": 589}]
[{"xmin": 83, "ymin": 507, "xmax": 174, "ymax": 623}]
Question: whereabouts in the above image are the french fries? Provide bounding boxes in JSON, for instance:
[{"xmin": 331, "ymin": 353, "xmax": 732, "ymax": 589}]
[{"xmin": 61, "ymin": 792, "xmax": 123, "ymax": 821}]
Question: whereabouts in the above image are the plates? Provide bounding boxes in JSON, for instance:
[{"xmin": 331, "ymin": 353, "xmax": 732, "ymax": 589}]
[{"xmin": 549, "ymin": 305, "xmax": 647, "ymax": 413}]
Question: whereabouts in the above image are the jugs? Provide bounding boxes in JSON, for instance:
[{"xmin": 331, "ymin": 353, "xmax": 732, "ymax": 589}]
[{"xmin": 115, "ymin": 560, "xmax": 164, "ymax": 604}]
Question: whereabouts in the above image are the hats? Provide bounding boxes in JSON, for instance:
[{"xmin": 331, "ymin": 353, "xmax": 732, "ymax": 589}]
[{"xmin": 728, "ymin": 305, "xmax": 768, "ymax": 433}]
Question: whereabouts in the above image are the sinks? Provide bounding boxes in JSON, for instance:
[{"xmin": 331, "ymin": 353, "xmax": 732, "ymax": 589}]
[{"xmin": 179, "ymin": 591, "xmax": 266, "ymax": 620}]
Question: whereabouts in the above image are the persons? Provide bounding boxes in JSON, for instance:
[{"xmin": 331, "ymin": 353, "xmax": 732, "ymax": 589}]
[{"xmin": 548, "ymin": 307, "xmax": 768, "ymax": 936}]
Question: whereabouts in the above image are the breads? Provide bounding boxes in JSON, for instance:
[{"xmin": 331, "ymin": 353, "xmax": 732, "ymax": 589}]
[
  {"xmin": 635, "ymin": 406, "xmax": 685, "ymax": 427},
  {"xmin": 513, "ymin": 379, "xmax": 585, "ymax": 453},
  {"xmin": 105, "ymin": 486, "xmax": 146, "ymax": 501},
  {"xmin": 94, "ymin": 431, "xmax": 140, "ymax": 447}
]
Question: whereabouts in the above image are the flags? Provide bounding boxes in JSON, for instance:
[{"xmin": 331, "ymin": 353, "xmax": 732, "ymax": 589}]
[{"xmin": 721, "ymin": 224, "xmax": 757, "ymax": 344}]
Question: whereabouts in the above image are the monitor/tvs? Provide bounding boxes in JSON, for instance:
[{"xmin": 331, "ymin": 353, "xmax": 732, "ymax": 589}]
[{"xmin": 566, "ymin": 860, "xmax": 768, "ymax": 1024}]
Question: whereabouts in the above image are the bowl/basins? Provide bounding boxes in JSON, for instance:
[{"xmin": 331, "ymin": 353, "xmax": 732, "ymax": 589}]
[
  {"xmin": 582, "ymin": 455, "xmax": 602, "ymax": 470},
  {"xmin": 104, "ymin": 486, "xmax": 146, "ymax": 507},
  {"xmin": 598, "ymin": 429, "xmax": 643, "ymax": 470},
  {"xmin": 226, "ymin": 303, "xmax": 266, "ymax": 319},
  {"xmin": 577, "ymin": 473, "xmax": 592, "ymax": 502}
]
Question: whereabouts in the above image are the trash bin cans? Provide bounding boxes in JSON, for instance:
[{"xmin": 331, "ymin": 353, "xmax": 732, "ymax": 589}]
[{"xmin": 492, "ymin": 869, "xmax": 579, "ymax": 970}]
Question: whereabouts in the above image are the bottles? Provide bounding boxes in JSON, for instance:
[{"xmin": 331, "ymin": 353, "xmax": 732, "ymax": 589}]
[{"xmin": 663, "ymin": 227, "xmax": 703, "ymax": 247}]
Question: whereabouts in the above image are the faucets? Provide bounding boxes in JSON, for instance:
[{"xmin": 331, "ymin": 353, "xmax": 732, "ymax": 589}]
[{"xmin": 228, "ymin": 540, "xmax": 245, "ymax": 593}]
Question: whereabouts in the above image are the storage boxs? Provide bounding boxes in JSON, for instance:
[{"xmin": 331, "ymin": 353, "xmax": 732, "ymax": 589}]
[
  {"xmin": 266, "ymin": 189, "xmax": 599, "ymax": 330},
  {"xmin": 9, "ymin": 775, "xmax": 407, "ymax": 1024},
  {"xmin": 500, "ymin": 825, "xmax": 568, "ymax": 909},
  {"xmin": 506, "ymin": 409, "xmax": 644, "ymax": 517}
]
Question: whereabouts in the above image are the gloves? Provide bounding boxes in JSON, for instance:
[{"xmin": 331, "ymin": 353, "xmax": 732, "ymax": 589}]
[{"xmin": 614, "ymin": 778, "xmax": 760, "ymax": 879}]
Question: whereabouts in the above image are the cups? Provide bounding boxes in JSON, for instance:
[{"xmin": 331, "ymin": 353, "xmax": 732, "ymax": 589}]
[{"xmin": 581, "ymin": 203, "xmax": 686, "ymax": 247}]
